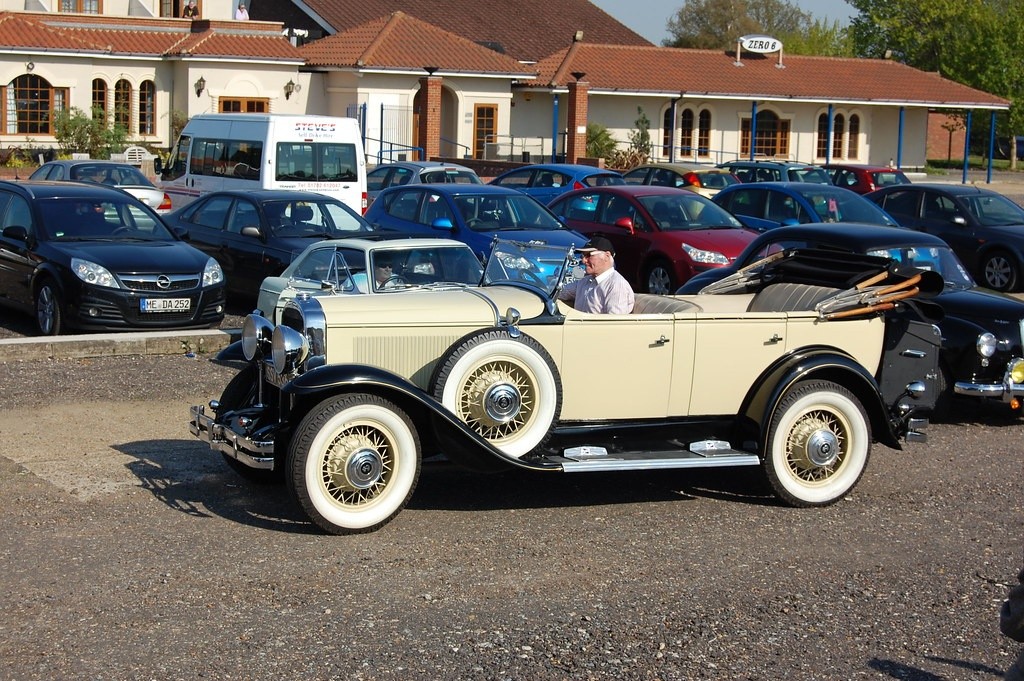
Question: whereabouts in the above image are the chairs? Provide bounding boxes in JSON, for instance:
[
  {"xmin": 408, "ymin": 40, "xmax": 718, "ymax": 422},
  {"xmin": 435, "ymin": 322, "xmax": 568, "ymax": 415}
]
[
  {"xmin": 798, "ymin": 197, "xmax": 816, "ymax": 222},
  {"xmin": 400, "ymin": 200, "xmax": 418, "ymax": 220},
  {"xmin": 927, "ymin": 201, "xmax": 940, "ymax": 220},
  {"xmin": 291, "ymin": 206, "xmax": 313, "ymax": 236},
  {"xmin": 737, "ymin": 172, "xmax": 749, "ymax": 183},
  {"xmin": 542, "ymin": 173, "xmax": 554, "ymax": 187},
  {"xmin": 757, "ymin": 169, "xmax": 766, "ymax": 181},
  {"xmin": 955, "ymin": 198, "xmax": 970, "ymax": 216},
  {"xmin": 709, "ymin": 175, "xmax": 724, "ymax": 186},
  {"xmin": 652, "ymin": 171, "xmax": 669, "ymax": 186},
  {"xmin": 653, "ymin": 201, "xmax": 670, "ymax": 229},
  {"xmin": 433, "ymin": 200, "xmax": 449, "ymax": 219},
  {"xmin": 763, "ymin": 174, "xmax": 774, "ymax": 180},
  {"xmin": 400, "ymin": 175, "xmax": 411, "ymax": 185}
]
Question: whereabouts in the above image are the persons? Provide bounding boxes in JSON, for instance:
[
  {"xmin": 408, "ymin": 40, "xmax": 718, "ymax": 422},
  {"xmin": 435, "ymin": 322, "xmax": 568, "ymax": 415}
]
[
  {"xmin": 546, "ymin": 236, "xmax": 635, "ymax": 315},
  {"xmin": 234, "ymin": 2, "xmax": 250, "ymax": 20},
  {"xmin": 183, "ymin": 0, "xmax": 199, "ymax": 20},
  {"xmin": 340, "ymin": 253, "xmax": 405, "ymax": 293}
]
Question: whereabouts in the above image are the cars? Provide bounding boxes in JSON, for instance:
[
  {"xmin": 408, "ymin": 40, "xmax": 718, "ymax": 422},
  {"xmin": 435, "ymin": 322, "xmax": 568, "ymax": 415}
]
[
  {"xmin": 0, "ymin": 180, "xmax": 226, "ymax": 335},
  {"xmin": 715, "ymin": 160, "xmax": 842, "ymax": 218},
  {"xmin": 840, "ymin": 183, "xmax": 1024, "ymax": 292},
  {"xmin": 697, "ymin": 181, "xmax": 901, "ymax": 248},
  {"xmin": 188, "ymin": 232, "xmax": 948, "ymax": 536},
  {"xmin": 674, "ymin": 223, "xmax": 1024, "ymax": 409},
  {"xmin": 161, "ymin": 189, "xmax": 442, "ymax": 297},
  {"xmin": 619, "ymin": 164, "xmax": 750, "ymax": 221},
  {"xmin": 366, "ymin": 161, "xmax": 485, "ymax": 202},
  {"xmin": 804, "ymin": 164, "xmax": 912, "ymax": 200},
  {"xmin": 359, "ymin": 183, "xmax": 590, "ymax": 290},
  {"xmin": 27, "ymin": 160, "xmax": 173, "ymax": 232},
  {"xmin": 543, "ymin": 185, "xmax": 784, "ymax": 295},
  {"xmin": 486, "ymin": 164, "xmax": 627, "ymax": 218}
]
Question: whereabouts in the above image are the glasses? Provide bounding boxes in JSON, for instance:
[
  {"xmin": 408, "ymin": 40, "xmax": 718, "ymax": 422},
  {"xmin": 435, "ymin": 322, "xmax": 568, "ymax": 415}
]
[{"xmin": 579, "ymin": 250, "xmax": 604, "ymax": 259}]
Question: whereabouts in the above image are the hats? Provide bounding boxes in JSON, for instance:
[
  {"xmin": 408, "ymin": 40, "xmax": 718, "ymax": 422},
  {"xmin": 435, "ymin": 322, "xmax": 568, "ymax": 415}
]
[{"xmin": 574, "ymin": 237, "xmax": 616, "ymax": 257}]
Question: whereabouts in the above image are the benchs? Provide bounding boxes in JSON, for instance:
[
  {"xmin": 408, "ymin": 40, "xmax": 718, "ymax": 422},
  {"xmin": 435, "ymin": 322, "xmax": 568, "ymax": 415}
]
[
  {"xmin": 625, "ymin": 293, "xmax": 706, "ymax": 315},
  {"xmin": 746, "ymin": 281, "xmax": 846, "ymax": 315}
]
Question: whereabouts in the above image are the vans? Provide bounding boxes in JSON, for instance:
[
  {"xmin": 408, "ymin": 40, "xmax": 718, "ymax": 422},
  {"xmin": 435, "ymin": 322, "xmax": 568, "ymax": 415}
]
[{"xmin": 154, "ymin": 112, "xmax": 367, "ymax": 232}]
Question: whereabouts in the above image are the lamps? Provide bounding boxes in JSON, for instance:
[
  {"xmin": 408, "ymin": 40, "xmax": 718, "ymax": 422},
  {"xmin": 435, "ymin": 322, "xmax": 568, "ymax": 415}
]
[
  {"xmin": 523, "ymin": 91, "xmax": 534, "ymax": 101},
  {"xmin": 196, "ymin": 76, "xmax": 207, "ymax": 98},
  {"xmin": 284, "ymin": 77, "xmax": 296, "ymax": 100}
]
